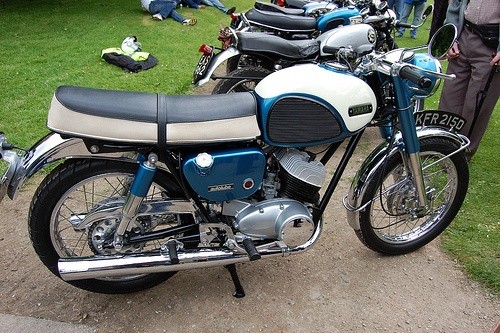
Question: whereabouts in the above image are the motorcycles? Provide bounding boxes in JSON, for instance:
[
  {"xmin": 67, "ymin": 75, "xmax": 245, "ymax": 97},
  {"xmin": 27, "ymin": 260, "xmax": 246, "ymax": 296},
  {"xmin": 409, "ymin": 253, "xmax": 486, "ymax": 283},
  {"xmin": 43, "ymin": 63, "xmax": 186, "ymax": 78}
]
[
  {"xmin": 230, "ymin": 0, "xmax": 380, "ymax": 40},
  {"xmin": 192, "ymin": 1, "xmax": 435, "ymax": 95},
  {"xmin": 0, "ymin": 23, "xmax": 471, "ymax": 299}
]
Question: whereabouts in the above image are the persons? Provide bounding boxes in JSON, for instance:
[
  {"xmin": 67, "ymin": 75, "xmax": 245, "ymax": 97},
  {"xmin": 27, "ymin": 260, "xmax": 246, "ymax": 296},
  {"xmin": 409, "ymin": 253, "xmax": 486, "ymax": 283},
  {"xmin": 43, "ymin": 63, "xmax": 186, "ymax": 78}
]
[
  {"xmin": 395, "ymin": 0, "xmax": 427, "ymax": 39},
  {"xmin": 184, "ymin": 1, "xmax": 236, "ymax": 15},
  {"xmin": 141, "ymin": 0, "xmax": 197, "ymax": 26},
  {"xmin": 443, "ymin": 0, "xmax": 500, "ymax": 160},
  {"xmin": 427, "ymin": 0, "xmax": 448, "ymax": 44}
]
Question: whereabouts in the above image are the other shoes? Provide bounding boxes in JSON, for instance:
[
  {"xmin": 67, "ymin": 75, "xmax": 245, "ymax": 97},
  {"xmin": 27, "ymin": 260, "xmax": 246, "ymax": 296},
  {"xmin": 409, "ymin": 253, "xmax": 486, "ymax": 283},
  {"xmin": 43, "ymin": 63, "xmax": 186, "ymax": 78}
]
[
  {"xmin": 226, "ymin": 5, "xmax": 236, "ymax": 14},
  {"xmin": 153, "ymin": 12, "xmax": 163, "ymax": 20},
  {"xmin": 198, "ymin": 4, "xmax": 205, "ymax": 8},
  {"xmin": 183, "ymin": 18, "xmax": 197, "ymax": 25},
  {"xmin": 410, "ymin": 35, "xmax": 416, "ymax": 38},
  {"xmin": 396, "ymin": 32, "xmax": 403, "ymax": 37}
]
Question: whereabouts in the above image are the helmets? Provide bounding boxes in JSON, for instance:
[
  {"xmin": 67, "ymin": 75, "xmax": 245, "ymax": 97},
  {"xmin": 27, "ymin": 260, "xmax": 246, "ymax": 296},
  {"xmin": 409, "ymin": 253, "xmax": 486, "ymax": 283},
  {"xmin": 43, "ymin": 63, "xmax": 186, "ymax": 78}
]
[{"xmin": 122, "ymin": 35, "xmax": 142, "ymax": 53}]
